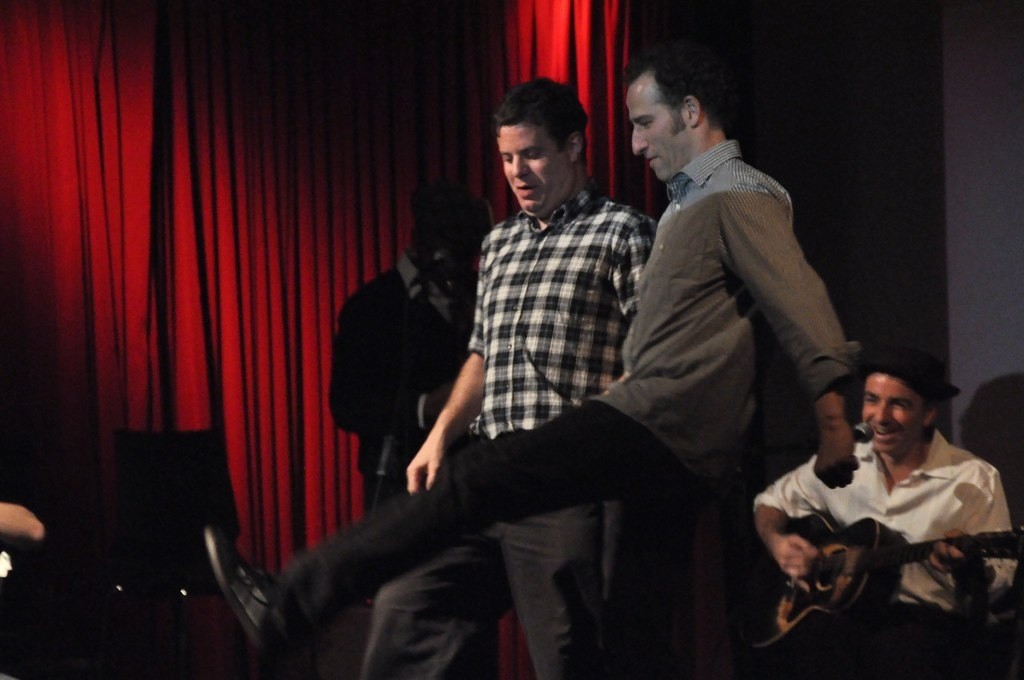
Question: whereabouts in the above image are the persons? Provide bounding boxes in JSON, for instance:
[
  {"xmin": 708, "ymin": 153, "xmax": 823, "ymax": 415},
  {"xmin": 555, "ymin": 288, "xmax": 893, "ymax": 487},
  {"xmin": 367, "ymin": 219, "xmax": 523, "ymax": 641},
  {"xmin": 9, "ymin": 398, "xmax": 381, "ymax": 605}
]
[
  {"xmin": 328, "ymin": 182, "xmax": 489, "ymax": 519},
  {"xmin": 205, "ymin": 51, "xmax": 867, "ymax": 680},
  {"xmin": 754, "ymin": 347, "xmax": 1018, "ymax": 679},
  {"xmin": 358, "ymin": 79, "xmax": 659, "ymax": 680}
]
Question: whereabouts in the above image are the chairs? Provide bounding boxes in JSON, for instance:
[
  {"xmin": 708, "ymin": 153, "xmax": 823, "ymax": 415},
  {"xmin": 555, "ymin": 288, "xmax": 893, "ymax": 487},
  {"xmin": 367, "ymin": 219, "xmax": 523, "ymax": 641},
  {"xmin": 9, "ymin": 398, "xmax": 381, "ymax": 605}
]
[{"xmin": 98, "ymin": 425, "xmax": 250, "ymax": 680}]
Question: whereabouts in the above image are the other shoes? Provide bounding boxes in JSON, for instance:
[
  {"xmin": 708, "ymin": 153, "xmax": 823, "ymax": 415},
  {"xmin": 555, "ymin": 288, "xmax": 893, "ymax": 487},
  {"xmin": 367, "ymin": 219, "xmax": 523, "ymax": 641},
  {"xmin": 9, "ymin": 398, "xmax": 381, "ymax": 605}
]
[{"xmin": 204, "ymin": 524, "xmax": 282, "ymax": 655}]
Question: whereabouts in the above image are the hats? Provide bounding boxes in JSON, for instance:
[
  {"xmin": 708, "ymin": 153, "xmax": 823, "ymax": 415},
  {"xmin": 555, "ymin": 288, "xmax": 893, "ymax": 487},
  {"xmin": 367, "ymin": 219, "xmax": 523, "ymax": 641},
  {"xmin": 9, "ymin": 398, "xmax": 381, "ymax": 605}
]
[
  {"xmin": 406, "ymin": 182, "xmax": 491, "ymax": 256},
  {"xmin": 843, "ymin": 348, "xmax": 961, "ymax": 401}
]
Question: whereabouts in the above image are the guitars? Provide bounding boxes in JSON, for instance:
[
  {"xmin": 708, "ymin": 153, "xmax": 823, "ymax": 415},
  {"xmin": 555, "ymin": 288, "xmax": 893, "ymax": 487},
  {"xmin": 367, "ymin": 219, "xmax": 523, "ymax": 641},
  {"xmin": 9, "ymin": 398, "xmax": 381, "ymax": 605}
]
[{"xmin": 735, "ymin": 510, "xmax": 1024, "ymax": 652}]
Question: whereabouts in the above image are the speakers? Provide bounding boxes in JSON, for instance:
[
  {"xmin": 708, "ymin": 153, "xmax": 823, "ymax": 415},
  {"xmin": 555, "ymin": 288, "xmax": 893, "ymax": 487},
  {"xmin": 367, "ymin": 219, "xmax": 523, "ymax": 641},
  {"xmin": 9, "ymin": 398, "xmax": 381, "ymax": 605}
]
[{"xmin": 116, "ymin": 430, "xmax": 236, "ymax": 602}]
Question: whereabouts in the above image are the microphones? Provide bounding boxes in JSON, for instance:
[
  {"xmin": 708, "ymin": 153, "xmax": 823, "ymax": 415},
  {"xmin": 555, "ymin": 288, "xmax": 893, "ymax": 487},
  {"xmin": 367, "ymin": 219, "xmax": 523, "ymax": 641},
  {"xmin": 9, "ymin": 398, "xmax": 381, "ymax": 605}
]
[
  {"xmin": 407, "ymin": 246, "xmax": 448, "ymax": 302},
  {"xmin": 851, "ymin": 423, "xmax": 874, "ymax": 443}
]
